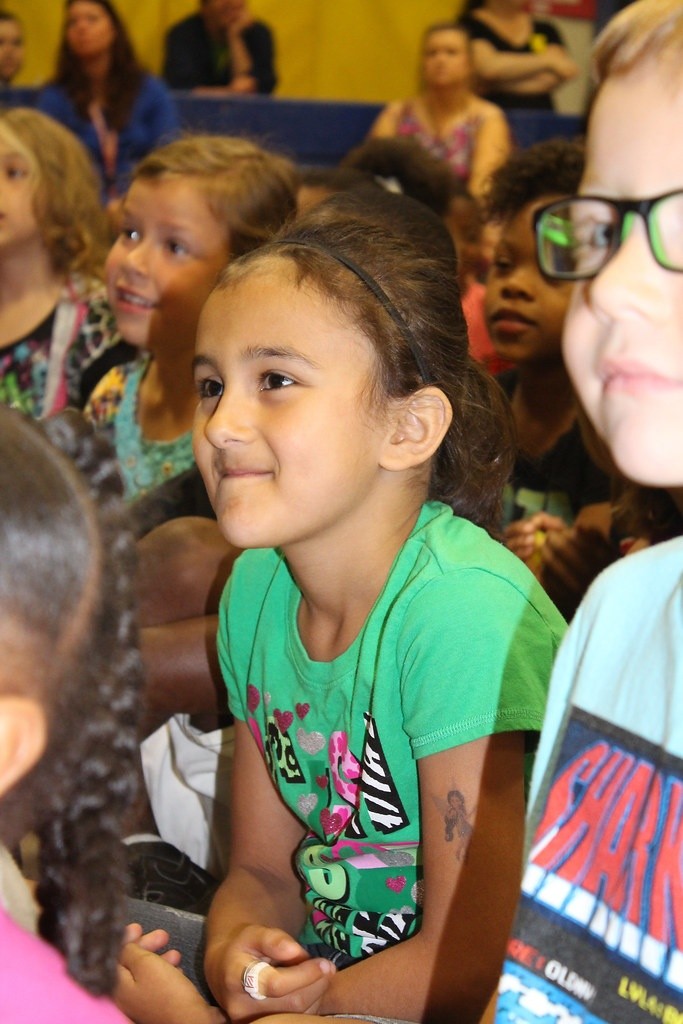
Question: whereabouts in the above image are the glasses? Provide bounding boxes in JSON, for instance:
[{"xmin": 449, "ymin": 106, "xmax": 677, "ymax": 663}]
[{"xmin": 533, "ymin": 189, "xmax": 683, "ymax": 282}]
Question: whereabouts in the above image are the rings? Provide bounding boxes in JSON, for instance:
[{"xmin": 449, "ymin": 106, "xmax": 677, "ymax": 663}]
[{"xmin": 243, "ymin": 959, "xmax": 272, "ymax": 1001}]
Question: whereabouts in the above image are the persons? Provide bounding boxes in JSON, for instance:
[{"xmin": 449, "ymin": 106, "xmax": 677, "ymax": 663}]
[
  {"xmin": 1, "ymin": 401, "xmax": 138, "ymax": 1021},
  {"xmin": 1, "ymin": 10, "xmax": 29, "ymax": 89},
  {"xmin": 292, "ymin": 1, "xmax": 607, "ymax": 515},
  {"xmin": 112, "ymin": 214, "xmax": 573, "ymax": 1024},
  {"xmin": 0, "ymin": 103, "xmax": 149, "ymax": 419},
  {"xmin": 156, "ymin": 0, "xmax": 280, "ymax": 97},
  {"xmin": 131, "ymin": 192, "xmax": 461, "ymax": 847},
  {"xmin": 475, "ymin": 1, "xmax": 682, "ymax": 1024},
  {"xmin": 78, "ymin": 132, "xmax": 302, "ymax": 502},
  {"xmin": 36, "ymin": 0, "xmax": 182, "ymax": 207}
]
